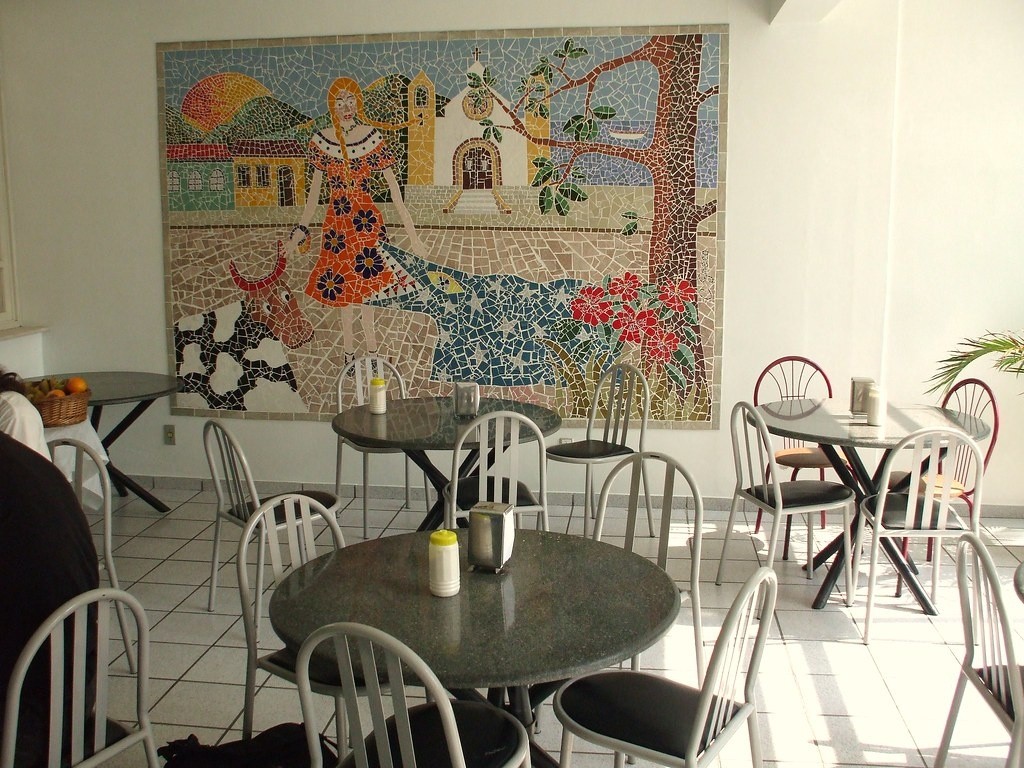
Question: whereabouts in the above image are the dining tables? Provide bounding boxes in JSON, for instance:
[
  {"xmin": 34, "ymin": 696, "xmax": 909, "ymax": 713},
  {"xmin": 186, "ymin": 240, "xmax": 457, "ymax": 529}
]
[
  {"xmin": 743, "ymin": 398, "xmax": 990, "ymax": 617},
  {"xmin": 330, "ymin": 393, "xmax": 563, "ymax": 536},
  {"xmin": 266, "ymin": 526, "xmax": 684, "ymax": 767},
  {"xmin": 9, "ymin": 371, "xmax": 188, "ymax": 512}
]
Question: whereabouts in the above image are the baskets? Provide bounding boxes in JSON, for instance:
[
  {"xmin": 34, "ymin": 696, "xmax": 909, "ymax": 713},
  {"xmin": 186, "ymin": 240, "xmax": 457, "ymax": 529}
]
[{"xmin": 19, "ymin": 381, "xmax": 92, "ymax": 428}]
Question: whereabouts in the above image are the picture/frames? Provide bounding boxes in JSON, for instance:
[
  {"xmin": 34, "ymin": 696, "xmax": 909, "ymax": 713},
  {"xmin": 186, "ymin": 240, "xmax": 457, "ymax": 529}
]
[{"xmin": 153, "ymin": 19, "xmax": 729, "ymax": 432}]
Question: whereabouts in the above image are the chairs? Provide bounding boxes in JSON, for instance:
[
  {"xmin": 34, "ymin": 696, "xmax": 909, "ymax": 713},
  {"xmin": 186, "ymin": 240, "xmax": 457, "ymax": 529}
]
[
  {"xmin": 552, "ymin": 566, "xmax": 782, "ymax": 767},
  {"xmin": 330, "ymin": 355, "xmax": 431, "ymax": 539},
  {"xmin": 235, "ymin": 491, "xmax": 396, "ymax": 768},
  {"xmin": 932, "ymin": 533, "xmax": 1024, "ymax": 767},
  {"xmin": 294, "ymin": 620, "xmax": 535, "ymax": 767},
  {"xmin": 715, "ymin": 400, "xmax": 857, "ymax": 619},
  {"xmin": 533, "ymin": 449, "xmax": 706, "ymax": 734},
  {"xmin": 851, "ymin": 426, "xmax": 985, "ymax": 648},
  {"xmin": 443, "ymin": 407, "xmax": 550, "ymax": 535},
  {"xmin": 199, "ymin": 419, "xmax": 343, "ymax": 640},
  {"xmin": 4, "ymin": 586, "xmax": 160, "ymax": 767},
  {"xmin": 866, "ymin": 376, "xmax": 1000, "ymax": 565},
  {"xmin": 752, "ymin": 354, "xmax": 861, "ymax": 544},
  {"xmin": 535, "ymin": 361, "xmax": 658, "ymax": 543},
  {"xmin": 36, "ymin": 439, "xmax": 142, "ymax": 673}
]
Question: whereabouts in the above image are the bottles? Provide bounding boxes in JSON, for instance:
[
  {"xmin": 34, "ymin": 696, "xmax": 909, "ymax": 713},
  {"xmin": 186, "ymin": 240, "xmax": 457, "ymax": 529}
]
[
  {"xmin": 866, "ymin": 384, "xmax": 887, "ymax": 427},
  {"xmin": 429, "ymin": 530, "xmax": 460, "ymax": 597},
  {"xmin": 369, "ymin": 378, "xmax": 386, "ymax": 414}
]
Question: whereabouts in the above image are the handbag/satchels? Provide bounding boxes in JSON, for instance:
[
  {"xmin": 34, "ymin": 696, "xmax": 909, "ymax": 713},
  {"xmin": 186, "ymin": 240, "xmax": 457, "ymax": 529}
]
[{"xmin": 156, "ymin": 720, "xmax": 340, "ymax": 767}]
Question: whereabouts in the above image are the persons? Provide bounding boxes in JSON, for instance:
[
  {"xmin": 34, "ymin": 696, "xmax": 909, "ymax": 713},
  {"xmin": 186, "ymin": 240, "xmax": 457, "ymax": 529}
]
[
  {"xmin": 0, "ymin": 368, "xmax": 53, "ymax": 465},
  {"xmin": 0, "ymin": 428, "xmax": 103, "ymax": 767}
]
